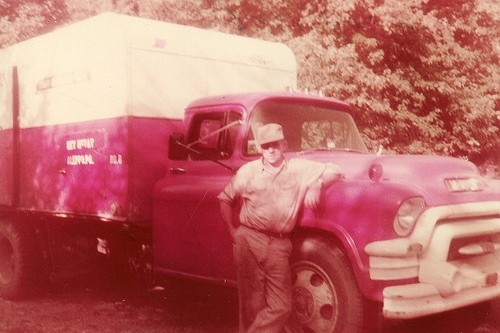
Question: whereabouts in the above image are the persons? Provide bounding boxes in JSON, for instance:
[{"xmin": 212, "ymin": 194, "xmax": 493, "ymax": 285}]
[{"xmin": 217, "ymin": 122, "xmax": 344, "ymax": 333}]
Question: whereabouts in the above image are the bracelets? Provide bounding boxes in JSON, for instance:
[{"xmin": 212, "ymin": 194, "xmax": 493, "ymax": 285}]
[{"xmin": 316, "ymin": 178, "xmax": 326, "ymax": 188}]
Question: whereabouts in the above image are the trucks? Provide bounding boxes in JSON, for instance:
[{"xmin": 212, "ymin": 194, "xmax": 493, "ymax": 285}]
[{"xmin": 1, "ymin": 12, "xmax": 500, "ymax": 333}]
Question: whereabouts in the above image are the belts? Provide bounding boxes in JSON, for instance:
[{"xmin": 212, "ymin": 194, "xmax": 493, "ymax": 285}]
[{"xmin": 241, "ymin": 221, "xmax": 291, "ymax": 242}]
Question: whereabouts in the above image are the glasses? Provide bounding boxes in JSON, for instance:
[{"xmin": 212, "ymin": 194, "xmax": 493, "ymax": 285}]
[{"xmin": 260, "ymin": 142, "xmax": 282, "ymax": 150}]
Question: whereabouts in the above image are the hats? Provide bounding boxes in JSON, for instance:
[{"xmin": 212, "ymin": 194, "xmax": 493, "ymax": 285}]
[{"xmin": 257, "ymin": 123, "xmax": 286, "ymax": 146}]
